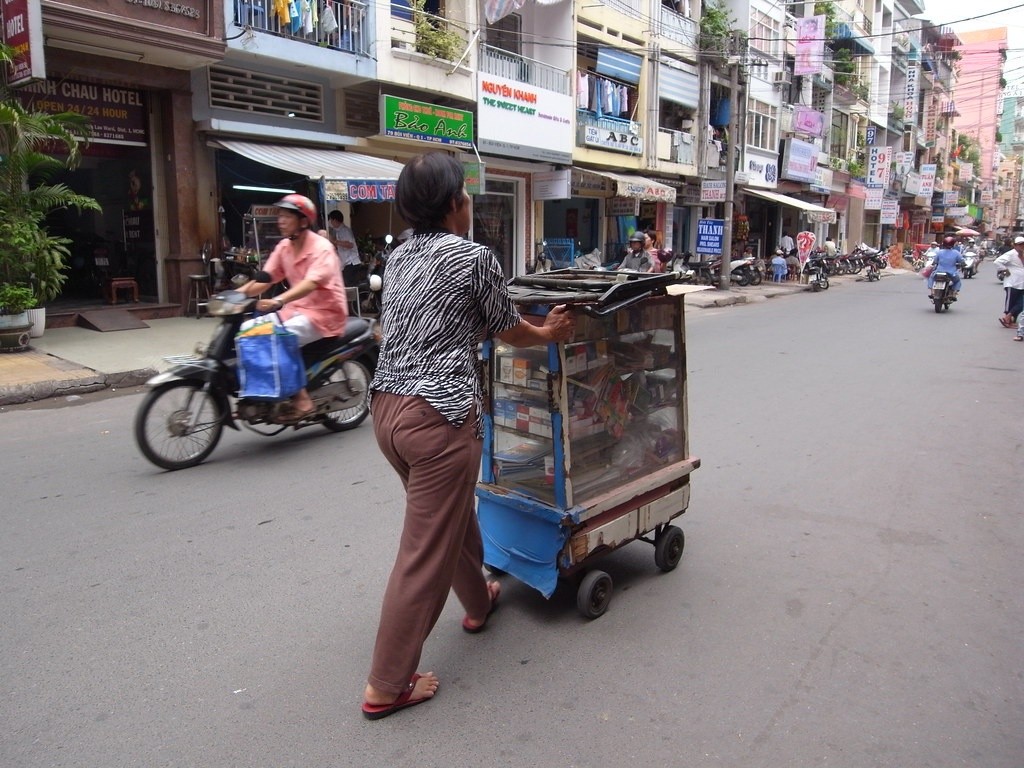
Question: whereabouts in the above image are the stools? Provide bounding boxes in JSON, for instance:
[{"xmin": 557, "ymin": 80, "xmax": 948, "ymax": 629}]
[
  {"xmin": 106, "ymin": 277, "xmax": 138, "ymax": 304},
  {"xmin": 186, "ymin": 274, "xmax": 211, "ymax": 318}
]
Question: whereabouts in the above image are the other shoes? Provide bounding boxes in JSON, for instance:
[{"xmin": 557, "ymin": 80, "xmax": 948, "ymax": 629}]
[
  {"xmin": 951, "ymin": 296, "xmax": 956, "ymax": 301},
  {"xmin": 1016, "ymin": 336, "xmax": 1023, "ymax": 340}
]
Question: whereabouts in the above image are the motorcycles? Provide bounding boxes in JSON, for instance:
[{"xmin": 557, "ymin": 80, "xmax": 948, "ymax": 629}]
[
  {"xmin": 132, "ymin": 254, "xmax": 383, "ymax": 471},
  {"xmin": 663, "ymin": 242, "xmax": 891, "ymax": 290},
  {"xmin": 928, "ymin": 262, "xmax": 968, "ymax": 314},
  {"xmin": 904, "ymin": 238, "xmax": 997, "ymax": 279}
]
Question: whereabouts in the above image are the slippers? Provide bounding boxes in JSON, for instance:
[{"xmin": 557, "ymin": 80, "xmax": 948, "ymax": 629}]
[
  {"xmin": 463, "ymin": 586, "xmax": 498, "ymax": 632},
  {"xmin": 999, "ymin": 318, "xmax": 1010, "ymax": 329},
  {"xmin": 363, "ymin": 673, "xmax": 438, "ymax": 720},
  {"xmin": 277, "ymin": 406, "xmax": 317, "ymax": 425}
]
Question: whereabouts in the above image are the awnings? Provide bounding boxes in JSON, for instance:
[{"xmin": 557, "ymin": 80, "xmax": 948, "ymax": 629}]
[
  {"xmin": 216, "ymin": 139, "xmax": 406, "ymax": 203},
  {"xmin": 830, "ymin": 22, "xmax": 875, "ymax": 57},
  {"xmin": 743, "ymin": 188, "xmax": 836, "ymax": 224},
  {"xmin": 572, "ymin": 165, "xmax": 676, "ymax": 204}
]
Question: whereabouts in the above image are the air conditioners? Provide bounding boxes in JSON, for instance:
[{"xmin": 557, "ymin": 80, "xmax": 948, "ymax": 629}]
[{"xmin": 773, "ymin": 71, "xmax": 793, "ymax": 85}]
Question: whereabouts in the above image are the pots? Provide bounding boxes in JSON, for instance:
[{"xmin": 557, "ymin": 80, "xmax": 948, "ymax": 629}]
[{"xmin": 210, "ymin": 258, "xmax": 224, "ymax": 279}]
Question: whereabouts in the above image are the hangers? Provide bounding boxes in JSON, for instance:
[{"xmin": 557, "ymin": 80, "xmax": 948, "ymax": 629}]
[{"xmin": 576, "ymin": 67, "xmax": 629, "ymax": 87}]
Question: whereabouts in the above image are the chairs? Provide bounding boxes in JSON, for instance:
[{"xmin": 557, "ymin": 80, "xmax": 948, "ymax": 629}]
[{"xmin": 757, "ymin": 262, "xmax": 798, "ymax": 281}]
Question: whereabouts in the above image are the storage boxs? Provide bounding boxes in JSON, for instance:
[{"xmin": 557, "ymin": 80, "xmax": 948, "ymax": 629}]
[{"xmin": 496, "ymin": 341, "xmax": 618, "ymax": 485}]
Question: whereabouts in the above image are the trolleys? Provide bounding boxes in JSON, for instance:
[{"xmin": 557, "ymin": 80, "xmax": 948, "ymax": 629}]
[{"xmin": 472, "ymin": 263, "xmax": 703, "ymax": 621}]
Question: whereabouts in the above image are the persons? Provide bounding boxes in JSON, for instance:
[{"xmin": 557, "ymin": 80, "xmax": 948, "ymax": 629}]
[
  {"xmin": 328, "ymin": 210, "xmax": 361, "ymax": 287},
  {"xmin": 824, "ymin": 237, "xmax": 835, "ymax": 257},
  {"xmin": 925, "ymin": 242, "xmax": 939, "ymax": 253},
  {"xmin": 964, "ymin": 239, "xmax": 979, "ymax": 259},
  {"xmin": 360, "ymin": 151, "xmax": 576, "ymax": 720},
  {"xmin": 772, "ymin": 250, "xmax": 787, "ymax": 275},
  {"xmin": 616, "ymin": 230, "xmax": 661, "ymax": 273},
  {"xmin": 994, "ymin": 236, "xmax": 1024, "ymax": 328},
  {"xmin": 785, "ymin": 249, "xmax": 801, "ymax": 273},
  {"xmin": 953, "ymin": 240, "xmax": 962, "ymax": 254},
  {"xmin": 781, "ymin": 231, "xmax": 794, "ymax": 255},
  {"xmin": 981, "ymin": 238, "xmax": 988, "ymax": 249},
  {"xmin": 928, "ymin": 236, "xmax": 966, "ymax": 301},
  {"xmin": 235, "ymin": 193, "xmax": 349, "ymax": 422},
  {"xmin": 999, "ymin": 238, "xmax": 1013, "ymax": 255}
]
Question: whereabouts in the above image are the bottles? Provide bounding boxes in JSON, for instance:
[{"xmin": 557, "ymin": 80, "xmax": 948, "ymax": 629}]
[{"xmin": 246, "ymin": 248, "xmax": 258, "ymax": 263}]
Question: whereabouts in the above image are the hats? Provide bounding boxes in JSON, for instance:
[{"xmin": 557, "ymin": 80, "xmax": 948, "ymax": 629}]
[
  {"xmin": 1014, "ymin": 236, "xmax": 1024, "ymax": 244},
  {"xmin": 776, "ymin": 250, "xmax": 783, "ymax": 255}
]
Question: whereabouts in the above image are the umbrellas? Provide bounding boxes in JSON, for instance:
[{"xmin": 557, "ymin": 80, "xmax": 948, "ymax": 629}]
[{"xmin": 956, "ymin": 228, "xmax": 979, "ymax": 236}]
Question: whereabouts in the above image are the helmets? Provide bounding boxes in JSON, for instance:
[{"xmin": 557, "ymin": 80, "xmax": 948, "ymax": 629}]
[
  {"xmin": 273, "ymin": 195, "xmax": 317, "ymax": 225},
  {"xmin": 931, "ymin": 241, "xmax": 937, "ymax": 246},
  {"xmin": 943, "ymin": 236, "xmax": 955, "ymax": 248},
  {"xmin": 969, "ymin": 239, "xmax": 975, "ymax": 244},
  {"xmin": 629, "ymin": 231, "xmax": 645, "ymax": 244}
]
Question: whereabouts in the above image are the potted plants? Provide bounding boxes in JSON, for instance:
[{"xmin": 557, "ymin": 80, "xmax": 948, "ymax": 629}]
[
  {"xmin": 0, "ymin": 94, "xmax": 101, "ymax": 339},
  {"xmin": 0, "ymin": 211, "xmax": 48, "ymax": 329}
]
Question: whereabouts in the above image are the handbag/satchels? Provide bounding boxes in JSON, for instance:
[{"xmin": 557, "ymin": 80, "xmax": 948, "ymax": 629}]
[{"xmin": 234, "ymin": 309, "xmax": 307, "ymax": 402}]
